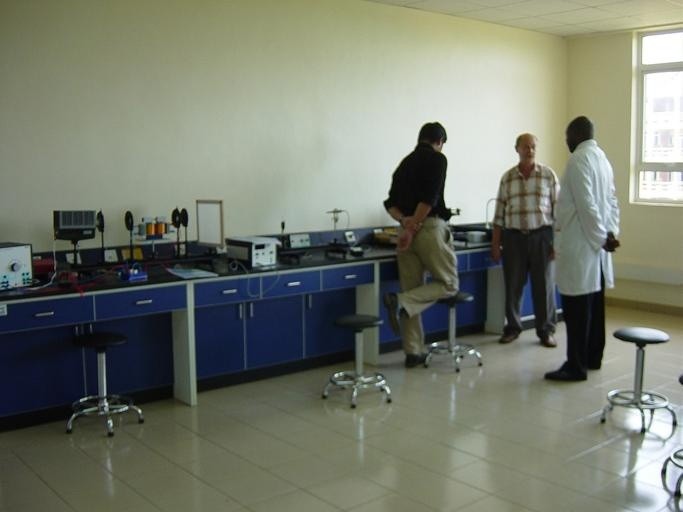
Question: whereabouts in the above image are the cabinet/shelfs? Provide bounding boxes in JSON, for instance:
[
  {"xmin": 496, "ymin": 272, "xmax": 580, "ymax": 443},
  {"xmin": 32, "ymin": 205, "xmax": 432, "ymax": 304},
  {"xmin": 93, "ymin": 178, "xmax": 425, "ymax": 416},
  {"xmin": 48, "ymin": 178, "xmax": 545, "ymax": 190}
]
[
  {"xmin": 193, "ymin": 260, "xmax": 379, "ymax": 403},
  {"xmin": 378, "ymin": 246, "xmax": 563, "ymax": 354},
  {"xmin": 0, "ymin": 281, "xmax": 192, "ymax": 428}
]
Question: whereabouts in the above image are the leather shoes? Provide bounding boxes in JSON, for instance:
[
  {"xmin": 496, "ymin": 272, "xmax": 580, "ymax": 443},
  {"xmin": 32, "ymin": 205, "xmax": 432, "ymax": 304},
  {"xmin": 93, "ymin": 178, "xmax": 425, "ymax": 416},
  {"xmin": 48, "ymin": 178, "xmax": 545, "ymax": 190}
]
[
  {"xmin": 499, "ymin": 329, "xmax": 519, "ymax": 343},
  {"xmin": 384, "ymin": 291, "xmax": 400, "ymax": 337},
  {"xmin": 544, "ymin": 358, "xmax": 601, "ymax": 382},
  {"xmin": 406, "ymin": 351, "xmax": 431, "ymax": 368},
  {"xmin": 542, "ymin": 330, "xmax": 557, "ymax": 347}
]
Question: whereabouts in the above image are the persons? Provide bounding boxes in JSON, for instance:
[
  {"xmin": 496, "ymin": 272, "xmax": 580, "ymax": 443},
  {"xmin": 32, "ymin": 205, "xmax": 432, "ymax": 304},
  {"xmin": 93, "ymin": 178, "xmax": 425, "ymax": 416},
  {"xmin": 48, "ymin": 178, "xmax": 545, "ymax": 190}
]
[
  {"xmin": 544, "ymin": 116, "xmax": 620, "ymax": 380},
  {"xmin": 489, "ymin": 133, "xmax": 562, "ymax": 345},
  {"xmin": 381, "ymin": 121, "xmax": 459, "ymax": 368}
]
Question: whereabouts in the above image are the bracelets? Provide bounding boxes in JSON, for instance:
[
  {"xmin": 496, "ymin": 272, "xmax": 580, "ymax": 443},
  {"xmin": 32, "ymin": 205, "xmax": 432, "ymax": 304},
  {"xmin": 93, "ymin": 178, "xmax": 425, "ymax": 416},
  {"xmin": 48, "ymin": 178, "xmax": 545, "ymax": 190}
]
[{"xmin": 398, "ymin": 214, "xmax": 404, "ymax": 224}]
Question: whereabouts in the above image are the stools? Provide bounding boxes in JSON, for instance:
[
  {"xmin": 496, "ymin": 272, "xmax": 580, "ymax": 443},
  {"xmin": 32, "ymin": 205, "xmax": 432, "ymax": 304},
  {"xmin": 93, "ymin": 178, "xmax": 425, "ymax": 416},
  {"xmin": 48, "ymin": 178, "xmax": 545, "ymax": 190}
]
[
  {"xmin": 321, "ymin": 314, "xmax": 395, "ymax": 408},
  {"xmin": 62, "ymin": 322, "xmax": 144, "ymax": 436},
  {"xmin": 663, "ymin": 370, "xmax": 683, "ymax": 498},
  {"xmin": 599, "ymin": 326, "xmax": 678, "ymax": 437},
  {"xmin": 421, "ymin": 291, "xmax": 483, "ymax": 374}
]
